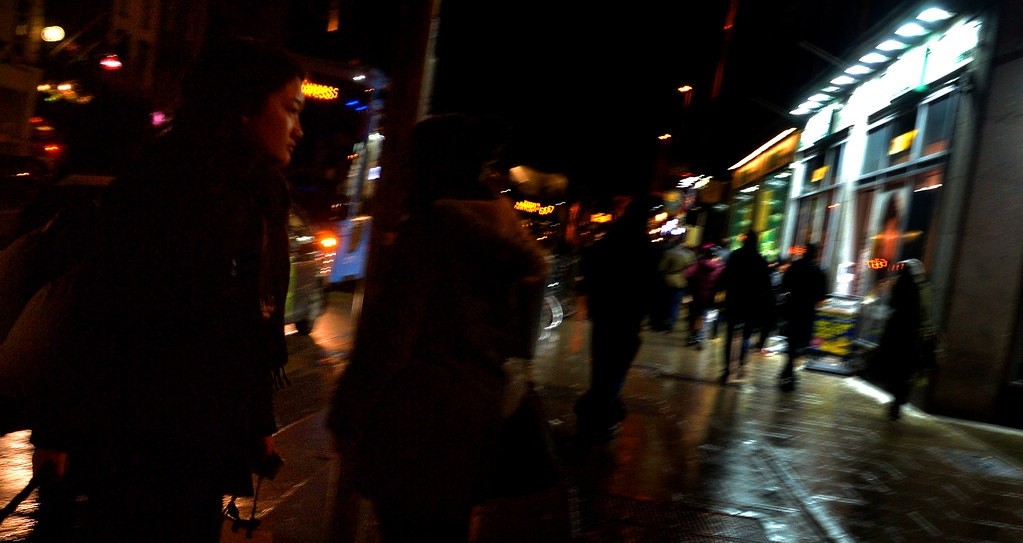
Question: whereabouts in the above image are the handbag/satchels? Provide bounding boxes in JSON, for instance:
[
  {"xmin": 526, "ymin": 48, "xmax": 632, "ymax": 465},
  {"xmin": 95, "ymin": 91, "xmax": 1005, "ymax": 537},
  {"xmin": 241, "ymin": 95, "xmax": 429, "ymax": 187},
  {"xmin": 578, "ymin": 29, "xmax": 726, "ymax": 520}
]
[
  {"xmin": 0, "ymin": 259, "xmax": 86, "ymax": 435},
  {"xmin": 487, "ymin": 391, "xmax": 573, "ymax": 502}
]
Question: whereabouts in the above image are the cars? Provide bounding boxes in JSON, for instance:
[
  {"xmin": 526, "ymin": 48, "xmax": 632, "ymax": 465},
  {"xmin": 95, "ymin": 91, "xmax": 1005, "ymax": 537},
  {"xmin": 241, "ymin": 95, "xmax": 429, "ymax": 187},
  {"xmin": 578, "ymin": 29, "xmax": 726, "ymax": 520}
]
[{"xmin": 278, "ymin": 210, "xmax": 326, "ymax": 334}]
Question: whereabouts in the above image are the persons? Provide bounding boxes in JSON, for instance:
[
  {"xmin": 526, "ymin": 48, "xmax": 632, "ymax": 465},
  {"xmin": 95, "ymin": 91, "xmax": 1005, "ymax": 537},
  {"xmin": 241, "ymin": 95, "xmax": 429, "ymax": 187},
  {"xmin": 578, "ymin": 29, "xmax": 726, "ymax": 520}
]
[
  {"xmin": 879, "ymin": 258, "xmax": 938, "ymax": 417},
  {"xmin": 1, "ymin": 36, "xmax": 304, "ymax": 543},
  {"xmin": 316, "ymin": 110, "xmax": 548, "ymax": 543},
  {"xmin": 651, "ymin": 229, "xmax": 828, "ymax": 390},
  {"xmin": 570, "ymin": 199, "xmax": 676, "ymax": 443}
]
